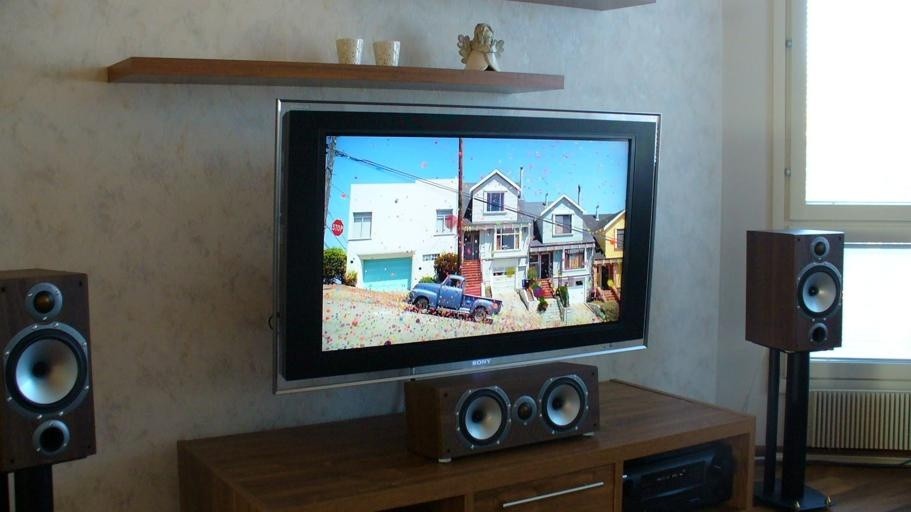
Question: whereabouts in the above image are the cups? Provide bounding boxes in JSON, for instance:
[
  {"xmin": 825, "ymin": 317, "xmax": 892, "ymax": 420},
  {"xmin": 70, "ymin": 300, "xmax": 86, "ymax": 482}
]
[
  {"xmin": 336, "ymin": 39, "xmax": 362, "ymax": 65},
  {"xmin": 372, "ymin": 40, "xmax": 401, "ymax": 67}
]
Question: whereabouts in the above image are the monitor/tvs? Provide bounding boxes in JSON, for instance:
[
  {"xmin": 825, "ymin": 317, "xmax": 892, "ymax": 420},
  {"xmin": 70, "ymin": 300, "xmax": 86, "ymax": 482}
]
[{"xmin": 272, "ymin": 98, "xmax": 661, "ymax": 393}]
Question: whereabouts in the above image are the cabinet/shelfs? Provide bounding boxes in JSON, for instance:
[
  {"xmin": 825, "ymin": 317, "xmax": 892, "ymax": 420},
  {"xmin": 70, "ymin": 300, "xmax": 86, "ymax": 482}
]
[{"xmin": 177, "ymin": 378, "xmax": 756, "ymax": 512}]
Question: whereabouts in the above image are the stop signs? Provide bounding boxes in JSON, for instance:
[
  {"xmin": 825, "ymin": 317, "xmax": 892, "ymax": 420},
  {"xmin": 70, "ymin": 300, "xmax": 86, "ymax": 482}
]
[{"xmin": 332, "ymin": 220, "xmax": 344, "ymax": 235}]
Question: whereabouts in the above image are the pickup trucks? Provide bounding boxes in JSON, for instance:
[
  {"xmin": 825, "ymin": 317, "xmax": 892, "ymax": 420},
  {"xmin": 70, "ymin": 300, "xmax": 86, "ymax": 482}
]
[{"xmin": 407, "ymin": 274, "xmax": 502, "ymax": 322}]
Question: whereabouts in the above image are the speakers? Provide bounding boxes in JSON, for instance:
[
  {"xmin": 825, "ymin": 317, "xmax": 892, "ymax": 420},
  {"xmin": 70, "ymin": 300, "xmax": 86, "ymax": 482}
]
[
  {"xmin": 0, "ymin": 270, "xmax": 97, "ymax": 473},
  {"xmin": 747, "ymin": 227, "xmax": 844, "ymax": 353},
  {"xmin": 403, "ymin": 362, "xmax": 600, "ymax": 461}
]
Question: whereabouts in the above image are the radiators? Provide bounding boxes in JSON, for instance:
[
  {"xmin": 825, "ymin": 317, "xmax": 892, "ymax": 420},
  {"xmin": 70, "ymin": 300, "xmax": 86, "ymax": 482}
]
[{"xmin": 810, "ymin": 389, "xmax": 911, "ymax": 450}]
